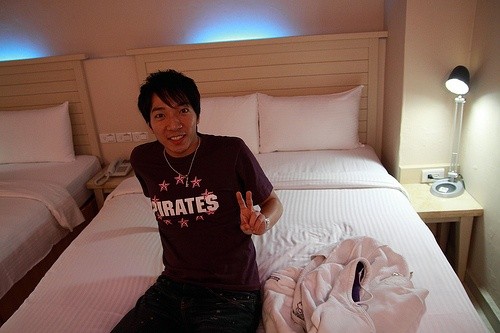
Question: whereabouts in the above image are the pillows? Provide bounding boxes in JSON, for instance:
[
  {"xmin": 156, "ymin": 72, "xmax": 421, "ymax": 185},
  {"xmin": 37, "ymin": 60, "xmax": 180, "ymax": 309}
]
[
  {"xmin": 258, "ymin": 85, "xmax": 364, "ymax": 154},
  {"xmin": 0, "ymin": 101, "xmax": 75, "ymax": 163},
  {"xmin": 197, "ymin": 93, "xmax": 259, "ymax": 155}
]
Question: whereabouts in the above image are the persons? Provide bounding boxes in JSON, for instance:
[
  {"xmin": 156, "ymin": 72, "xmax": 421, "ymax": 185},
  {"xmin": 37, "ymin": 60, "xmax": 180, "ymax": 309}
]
[{"xmin": 110, "ymin": 69, "xmax": 284, "ymax": 333}]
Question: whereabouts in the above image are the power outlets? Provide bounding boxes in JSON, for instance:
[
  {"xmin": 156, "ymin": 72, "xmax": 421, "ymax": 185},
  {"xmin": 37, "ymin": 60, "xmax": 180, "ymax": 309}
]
[{"xmin": 421, "ymin": 168, "xmax": 445, "ymax": 184}]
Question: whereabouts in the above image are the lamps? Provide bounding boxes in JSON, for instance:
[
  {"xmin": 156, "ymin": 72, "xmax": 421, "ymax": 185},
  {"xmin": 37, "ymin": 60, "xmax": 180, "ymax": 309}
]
[{"xmin": 430, "ymin": 65, "xmax": 470, "ymax": 199}]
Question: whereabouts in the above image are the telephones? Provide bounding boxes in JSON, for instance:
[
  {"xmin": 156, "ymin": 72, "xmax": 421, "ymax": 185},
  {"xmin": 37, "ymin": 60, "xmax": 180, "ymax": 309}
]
[{"xmin": 105, "ymin": 158, "xmax": 133, "ymax": 176}]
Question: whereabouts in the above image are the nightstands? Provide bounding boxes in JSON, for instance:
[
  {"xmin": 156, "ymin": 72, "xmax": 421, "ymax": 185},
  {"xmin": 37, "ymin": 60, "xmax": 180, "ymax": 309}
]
[
  {"xmin": 85, "ymin": 164, "xmax": 136, "ymax": 212},
  {"xmin": 401, "ymin": 183, "xmax": 484, "ymax": 283}
]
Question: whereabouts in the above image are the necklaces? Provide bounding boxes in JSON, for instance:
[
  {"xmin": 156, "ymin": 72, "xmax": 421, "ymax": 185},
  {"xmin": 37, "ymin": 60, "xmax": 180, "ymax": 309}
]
[{"xmin": 163, "ymin": 135, "xmax": 201, "ymax": 187}]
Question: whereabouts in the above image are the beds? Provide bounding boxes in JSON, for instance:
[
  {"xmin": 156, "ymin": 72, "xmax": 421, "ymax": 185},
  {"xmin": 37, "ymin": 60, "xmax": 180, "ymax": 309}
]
[
  {"xmin": 0, "ymin": 154, "xmax": 102, "ymax": 328},
  {"xmin": 0, "ymin": 143, "xmax": 491, "ymax": 333}
]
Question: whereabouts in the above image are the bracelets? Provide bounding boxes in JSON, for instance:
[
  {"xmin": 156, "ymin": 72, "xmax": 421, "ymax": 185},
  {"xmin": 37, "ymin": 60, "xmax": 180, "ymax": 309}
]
[{"xmin": 263, "ymin": 215, "xmax": 270, "ymax": 230}]
